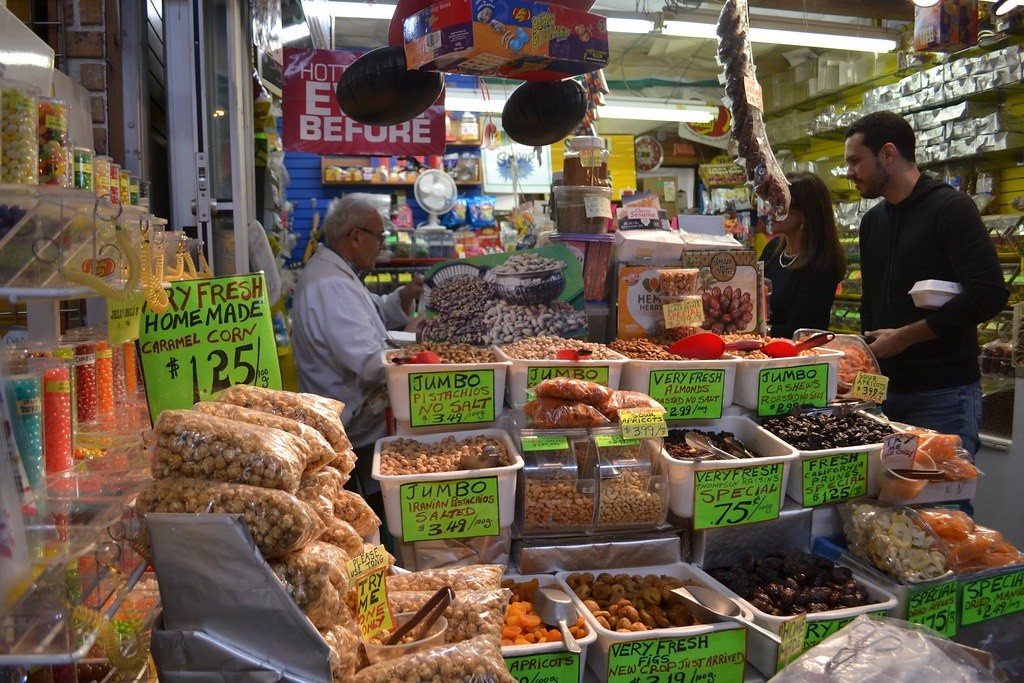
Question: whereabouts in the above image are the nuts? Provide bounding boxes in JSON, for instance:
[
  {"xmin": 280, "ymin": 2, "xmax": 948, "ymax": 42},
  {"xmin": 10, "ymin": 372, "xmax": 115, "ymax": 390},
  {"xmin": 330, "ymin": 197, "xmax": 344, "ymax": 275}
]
[
  {"xmin": 388, "ymin": 333, "xmax": 816, "ymax": 365},
  {"xmin": 424, "ymin": 252, "xmax": 589, "ymax": 343},
  {"xmin": 381, "ymin": 433, "xmax": 665, "ymax": 528}
]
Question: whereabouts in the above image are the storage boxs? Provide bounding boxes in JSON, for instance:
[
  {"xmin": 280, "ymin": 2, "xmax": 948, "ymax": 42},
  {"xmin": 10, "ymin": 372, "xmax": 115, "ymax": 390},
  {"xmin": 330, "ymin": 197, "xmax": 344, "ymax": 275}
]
[
  {"xmin": 613, "ymin": 229, "xmax": 685, "ymax": 261},
  {"xmin": 370, "ymin": 345, "xmax": 1024, "ymax": 683},
  {"xmin": 680, "ymin": 232, "xmax": 743, "ymax": 260}
]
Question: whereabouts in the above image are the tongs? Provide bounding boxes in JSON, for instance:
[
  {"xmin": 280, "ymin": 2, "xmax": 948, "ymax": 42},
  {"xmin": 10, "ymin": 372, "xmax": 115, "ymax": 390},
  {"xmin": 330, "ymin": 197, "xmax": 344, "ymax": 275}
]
[
  {"xmin": 886, "ymin": 465, "xmax": 947, "ymax": 478},
  {"xmin": 685, "ymin": 431, "xmax": 753, "ymax": 460},
  {"xmin": 791, "ymin": 399, "xmax": 877, "ymax": 418},
  {"xmin": 381, "ymin": 584, "xmax": 460, "ymax": 658}
]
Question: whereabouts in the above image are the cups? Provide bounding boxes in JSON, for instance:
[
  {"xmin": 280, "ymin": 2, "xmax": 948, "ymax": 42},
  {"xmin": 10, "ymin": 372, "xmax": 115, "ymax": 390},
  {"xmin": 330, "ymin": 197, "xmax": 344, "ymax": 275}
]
[{"xmin": 877, "ymin": 447, "xmax": 936, "ymax": 500}]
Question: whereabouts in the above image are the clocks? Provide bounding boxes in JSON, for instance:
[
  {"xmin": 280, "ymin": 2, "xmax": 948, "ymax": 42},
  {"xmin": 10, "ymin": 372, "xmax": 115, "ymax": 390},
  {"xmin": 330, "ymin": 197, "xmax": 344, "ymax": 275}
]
[{"xmin": 635, "ymin": 135, "xmax": 664, "ymax": 173}]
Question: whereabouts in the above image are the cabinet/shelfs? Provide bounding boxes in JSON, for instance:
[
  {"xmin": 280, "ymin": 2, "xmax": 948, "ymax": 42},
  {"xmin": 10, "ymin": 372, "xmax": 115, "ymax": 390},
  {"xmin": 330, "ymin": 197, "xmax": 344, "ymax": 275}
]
[
  {"xmin": 762, "ymin": 32, "xmax": 1024, "ymax": 334},
  {"xmin": 0, "ymin": 184, "xmax": 206, "ymax": 683}
]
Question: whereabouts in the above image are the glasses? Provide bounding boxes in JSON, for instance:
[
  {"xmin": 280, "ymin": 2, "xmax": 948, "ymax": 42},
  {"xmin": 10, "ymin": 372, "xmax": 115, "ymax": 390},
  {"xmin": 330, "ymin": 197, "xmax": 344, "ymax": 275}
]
[{"xmin": 345, "ymin": 226, "xmax": 386, "ymax": 243}]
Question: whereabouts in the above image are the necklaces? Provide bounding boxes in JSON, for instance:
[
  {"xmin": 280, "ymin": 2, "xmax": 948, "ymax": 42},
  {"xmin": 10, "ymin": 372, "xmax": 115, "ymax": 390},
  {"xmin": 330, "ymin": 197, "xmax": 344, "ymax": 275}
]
[{"xmin": 779, "ymin": 250, "xmax": 801, "ymax": 266}]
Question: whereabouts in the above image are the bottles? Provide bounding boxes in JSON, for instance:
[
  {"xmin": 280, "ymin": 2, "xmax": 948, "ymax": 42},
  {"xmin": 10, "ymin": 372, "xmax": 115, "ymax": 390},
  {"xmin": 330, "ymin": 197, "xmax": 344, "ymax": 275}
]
[
  {"xmin": 0, "ymin": 62, "xmax": 152, "ymax": 215},
  {"xmin": 562, "ymin": 136, "xmax": 609, "ymax": 187},
  {"xmin": 444, "ymin": 111, "xmax": 480, "ymax": 181},
  {"xmin": 0, "ymin": 321, "xmax": 145, "ymax": 490},
  {"xmin": 920, "ymin": 162, "xmax": 1002, "ymax": 215}
]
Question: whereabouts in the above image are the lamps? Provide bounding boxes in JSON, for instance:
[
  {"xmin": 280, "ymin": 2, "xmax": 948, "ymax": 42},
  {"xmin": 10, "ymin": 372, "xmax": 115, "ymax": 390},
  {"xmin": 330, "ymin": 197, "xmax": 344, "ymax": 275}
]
[
  {"xmin": 301, "ymin": 0, "xmax": 663, "ymax": 38},
  {"xmin": 991, "ymin": 0, "xmax": 1024, "ymax": 16},
  {"xmin": 660, "ymin": 4, "xmax": 905, "ymax": 54},
  {"xmin": 444, "ymin": 88, "xmax": 721, "ymax": 124},
  {"xmin": 912, "ymin": 0, "xmax": 940, "ymax": 7},
  {"xmin": 280, "ymin": 3, "xmax": 309, "ymax": 45}
]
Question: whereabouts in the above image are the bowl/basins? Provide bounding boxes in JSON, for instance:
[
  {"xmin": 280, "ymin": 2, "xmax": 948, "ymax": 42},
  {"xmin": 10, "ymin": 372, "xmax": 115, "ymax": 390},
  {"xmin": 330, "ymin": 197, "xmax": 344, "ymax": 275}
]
[
  {"xmin": 358, "ymin": 611, "xmax": 449, "ymax": 666},
  {"xmin": 907, "ymin": 279, "xmax": 964, "ymax": 311},
  {"xmin": 551, "ymin": 185, "xmax": 614, "ymax": 235}
]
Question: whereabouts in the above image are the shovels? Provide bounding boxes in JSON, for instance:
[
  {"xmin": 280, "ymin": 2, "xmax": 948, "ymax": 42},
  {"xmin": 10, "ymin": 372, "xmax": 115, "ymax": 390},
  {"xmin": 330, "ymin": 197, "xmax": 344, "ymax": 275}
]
[
  {"xmin": 392, "ymin": 351, "xmax": 441, "ymax": 364},
  {"xmin": 670, "ymin": 333, "xmax": 764, "ymax": 360},
  {"xmin": 670, "ymin": 586, "xmax": 782, "ymax": 644},
  {"xmin": 760, "ymin": 333, "xmax": 835, "ymax": 358},
  {"xmin": 535, "ymin": 588, "xmax": 581, "ymax": 654},
  {"xmin": 557, "ymin": 349, "xmax": 592, "ymax": 360}
]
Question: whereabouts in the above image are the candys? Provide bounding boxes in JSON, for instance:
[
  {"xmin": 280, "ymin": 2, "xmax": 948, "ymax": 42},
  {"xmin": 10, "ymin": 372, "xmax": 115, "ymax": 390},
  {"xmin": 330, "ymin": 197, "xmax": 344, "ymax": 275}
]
[
  {"xmin": 46, "ymin": 450, "xmax": 130, "ymax": 539},
  {"xmin": 2, "ymin": 334, "xmax": 138, "ymax": 491},
  {"xmin": 43, "ymin": 549, "xmax": 163, "ymax": 683},
  {"xmin": 0, "ymin": 82, "xmax": 151, "ymax": 208}
]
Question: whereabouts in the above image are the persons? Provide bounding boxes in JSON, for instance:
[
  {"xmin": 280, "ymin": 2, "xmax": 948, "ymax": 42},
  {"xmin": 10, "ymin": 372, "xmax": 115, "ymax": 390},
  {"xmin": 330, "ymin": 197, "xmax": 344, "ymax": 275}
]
[
  {"xmin": 291, "ymin": 197, "xmax": 424, "ymax": 554},
  {"xmin": 758, "ymin": 171, "xmax": 847, "ymax": 339},
  {"xmin": 844, "ymin": 110, "xmax": 1011, "ymax": 517}
]
